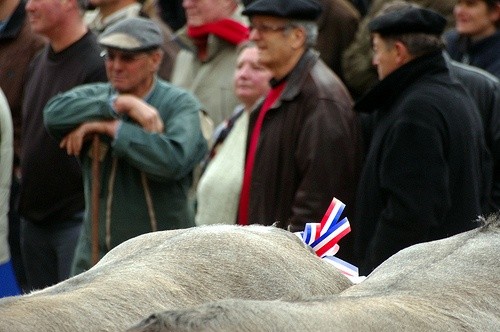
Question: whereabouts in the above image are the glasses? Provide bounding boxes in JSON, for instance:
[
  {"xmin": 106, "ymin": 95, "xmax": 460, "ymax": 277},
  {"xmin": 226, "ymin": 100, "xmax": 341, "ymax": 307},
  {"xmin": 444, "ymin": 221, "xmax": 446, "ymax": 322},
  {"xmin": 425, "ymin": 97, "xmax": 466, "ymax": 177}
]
[
  {"xmin": 100, "ymin": 51, "xmax": 156, "ymax": 64},
  {"xmin": 251, "ymin": 22, "xmax": 292, "ymax": 34}
]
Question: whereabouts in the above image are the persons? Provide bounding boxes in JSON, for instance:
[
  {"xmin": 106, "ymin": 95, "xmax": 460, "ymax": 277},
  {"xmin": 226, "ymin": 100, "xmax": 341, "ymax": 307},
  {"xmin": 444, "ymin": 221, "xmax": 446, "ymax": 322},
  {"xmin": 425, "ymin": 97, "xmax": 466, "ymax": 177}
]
[
  {"xmin": 0, "ymin": 87, "xmax": 19, "ymax": 299},
  {"xmin": 0, "ymin": 0, "xmax": 110, "ymax": 294},
  {"xmin": 41, "ymin": 18, "xmax": 208, "ymax": 279},
  {"xmin": 82, "ymin": 0, "xmax": 500, "ymax": 225},
  {"xmin": 350, "ymin": 7, "xmax": 493, "ymax": 275},
  {"xmin": 193, "ymin": 0, "xmax": 362, "ymax": 233}
]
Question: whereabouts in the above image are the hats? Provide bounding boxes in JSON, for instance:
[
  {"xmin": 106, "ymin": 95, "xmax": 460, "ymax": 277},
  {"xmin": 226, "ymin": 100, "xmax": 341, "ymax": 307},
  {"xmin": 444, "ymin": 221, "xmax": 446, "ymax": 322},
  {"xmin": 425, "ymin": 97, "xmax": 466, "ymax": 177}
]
[
  {"xmin": 241, "ymin": 0, "xmax": 322, "ymax": 21},
  {"xmin": 368, "ymin": 3, "xmax": 448, "ymax": 35},
  {"xmin": 96, "ymin": 17, "xmax": 163, "ymax": 50}
]
